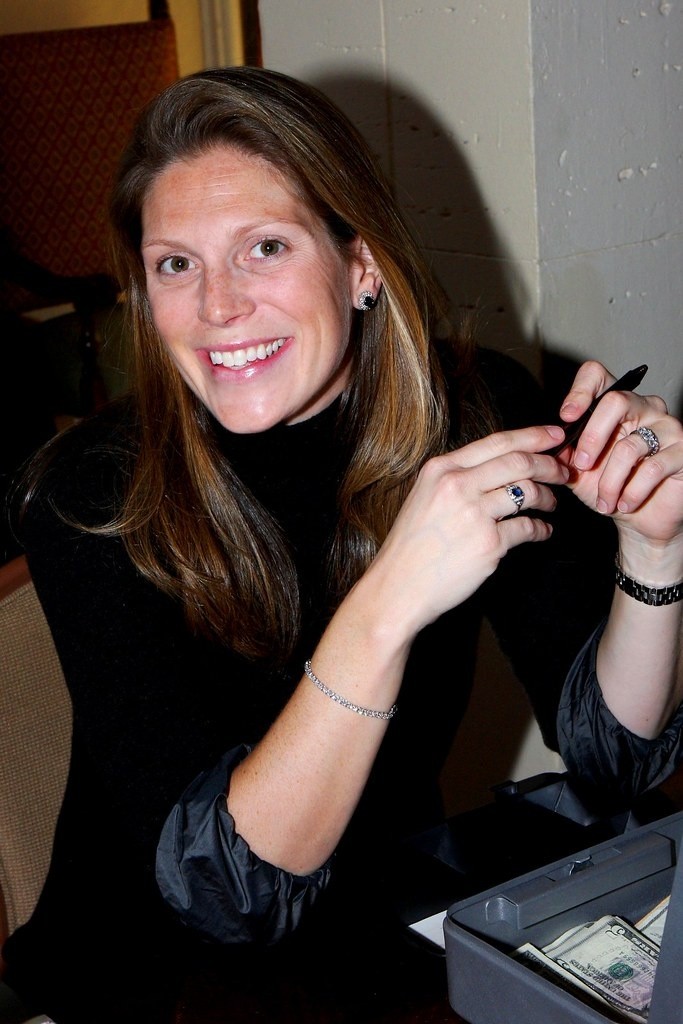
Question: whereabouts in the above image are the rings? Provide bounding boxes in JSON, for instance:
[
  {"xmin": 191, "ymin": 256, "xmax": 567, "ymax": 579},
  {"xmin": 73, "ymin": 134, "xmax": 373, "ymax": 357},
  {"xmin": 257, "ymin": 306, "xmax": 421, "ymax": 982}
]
[
  {"xmin": 507, "ymin": 483, "xmax": 524, "ymax": 515},
  {"xmin": 629, "ymin": 426, "xmax": 659, "ymax": 459}
]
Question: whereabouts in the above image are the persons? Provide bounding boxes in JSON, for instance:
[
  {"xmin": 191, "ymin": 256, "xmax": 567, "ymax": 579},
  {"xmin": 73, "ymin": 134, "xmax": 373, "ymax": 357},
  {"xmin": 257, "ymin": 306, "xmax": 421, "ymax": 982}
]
[{"xmin": 3, "ymin": 62, "xmax": 682, "ymax": 1024}]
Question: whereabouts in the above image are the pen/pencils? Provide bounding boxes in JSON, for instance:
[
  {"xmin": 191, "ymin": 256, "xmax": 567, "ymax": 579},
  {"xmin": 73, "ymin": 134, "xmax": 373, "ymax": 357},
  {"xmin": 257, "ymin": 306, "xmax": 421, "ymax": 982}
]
[{"xmin": 532, "ymin": 365, "xmax": 650, "ymax": 461}]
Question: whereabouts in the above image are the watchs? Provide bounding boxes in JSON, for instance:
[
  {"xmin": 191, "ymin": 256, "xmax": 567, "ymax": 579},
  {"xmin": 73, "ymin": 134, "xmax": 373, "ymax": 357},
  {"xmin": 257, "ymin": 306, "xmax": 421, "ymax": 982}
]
[{"xmin": 610, "ymin": 549, "xmax": 682, "ymax": 608}]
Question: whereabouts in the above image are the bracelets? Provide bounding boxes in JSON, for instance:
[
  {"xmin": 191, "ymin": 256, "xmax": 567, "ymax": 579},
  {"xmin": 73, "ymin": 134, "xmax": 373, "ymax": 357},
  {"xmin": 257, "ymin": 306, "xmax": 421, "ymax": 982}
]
[{"xmin": 302, "ymin": 660, "xmax": 398, "ymax": 721}]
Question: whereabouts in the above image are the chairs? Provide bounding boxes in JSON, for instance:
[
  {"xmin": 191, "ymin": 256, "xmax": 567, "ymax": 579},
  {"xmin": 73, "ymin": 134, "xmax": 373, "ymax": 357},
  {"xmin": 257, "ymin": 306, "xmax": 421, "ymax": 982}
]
[{"xmin": 0, "ymin": 558, "xmax": 74, "ymax": 942}]
